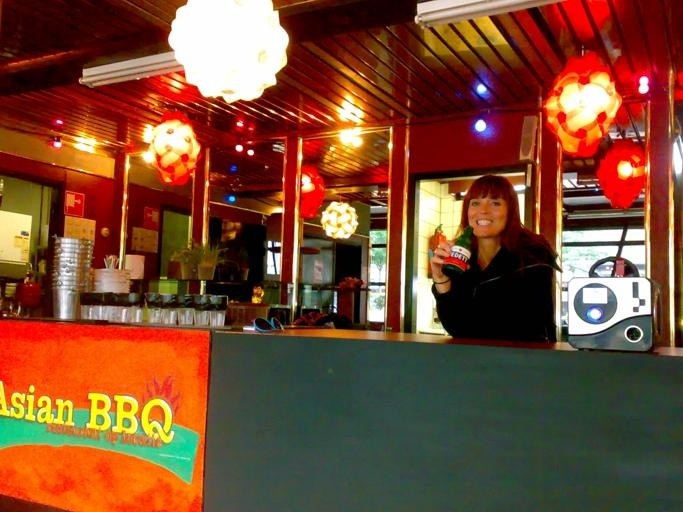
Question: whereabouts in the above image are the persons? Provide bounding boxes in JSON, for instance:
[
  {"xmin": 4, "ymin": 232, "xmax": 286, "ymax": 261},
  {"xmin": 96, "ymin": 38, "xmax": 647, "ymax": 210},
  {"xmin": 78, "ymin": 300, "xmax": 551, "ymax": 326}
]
[{"xmin": 429, "ymin": 173, "xmax": 564, "ymax": 342}]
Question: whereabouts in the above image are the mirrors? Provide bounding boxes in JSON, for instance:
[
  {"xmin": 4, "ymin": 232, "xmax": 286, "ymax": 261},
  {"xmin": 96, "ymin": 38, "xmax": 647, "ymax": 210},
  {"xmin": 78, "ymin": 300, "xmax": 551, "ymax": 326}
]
[
  {"xmin": 199, "ymin": 134, "xmax": 287, "ymax": 303},
  {"xmin": 118, "ymin": 151, "xmax": 198, "ymax": 279},
  {"xmin": 291, "ymin": 129, "xmax": 394, "ymax": 327},
  {"xmin": 555, "ymin": 102, "xmax": 651, "ymax": 347},
  {"xmin": 669, "ymin": 70, "xmax": 683, "ymax": 349}
]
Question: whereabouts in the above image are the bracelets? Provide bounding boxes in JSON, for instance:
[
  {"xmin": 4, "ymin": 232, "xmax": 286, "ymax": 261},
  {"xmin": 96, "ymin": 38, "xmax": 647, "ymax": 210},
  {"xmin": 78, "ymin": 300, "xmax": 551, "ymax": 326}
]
[{"xmin": 433, "ymin": 276, "xmax": 451, "ymax": 286}]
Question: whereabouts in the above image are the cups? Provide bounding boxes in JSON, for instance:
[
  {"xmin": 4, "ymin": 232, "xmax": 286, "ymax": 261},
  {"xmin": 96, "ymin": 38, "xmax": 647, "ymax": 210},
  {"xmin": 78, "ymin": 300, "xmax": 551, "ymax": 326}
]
[
  {"xmin": 192, "ymin": 294, "xmax": 210, "ymax": 326},
  {"xmin": 175, "ymin": 295, "xmax": 193, "ymax": 325},
  {"xmin": 80, "ymin": 293, "xmax": 129, "ymax": 320},
  {"xmin": 146, "ymin": 293, "xmax": 161, "ymax": 324},
  {"xmin": 208, "ymin": 296, "xmax": 228, "ymax": 326},
  {"xmin": 161, "ymin": 295, "xmax": 177, "ymax": 324},
  {"xmin": 128, "ymin": 293, "xmax": 144, "ymax": 322}
]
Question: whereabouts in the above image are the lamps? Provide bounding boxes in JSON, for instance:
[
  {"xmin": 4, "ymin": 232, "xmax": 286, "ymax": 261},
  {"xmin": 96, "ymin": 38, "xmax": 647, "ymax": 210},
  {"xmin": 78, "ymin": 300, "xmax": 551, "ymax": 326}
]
[
  {"xmin": 153, "ymin": 109, "xmax": 201, "ymax": 187},
  {"xmin": 321, "ymin": 200, "xmax": 360, "ymax": 238},
  {"xmin": 168, "ymin": 0, "xmax": 289, "ymax": 103},
  {"xmin": 542, "ymin": 47, "xmax": 622, "ymax": 159},
  {"xmin": 598, "ymin": 141, "xmax": 649, "ymax": 208}
]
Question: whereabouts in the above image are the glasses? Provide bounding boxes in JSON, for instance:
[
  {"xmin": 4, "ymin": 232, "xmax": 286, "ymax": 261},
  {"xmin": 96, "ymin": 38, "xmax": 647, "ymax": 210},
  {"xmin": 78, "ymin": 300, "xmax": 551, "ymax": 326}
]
[{"xmin": 254, "ymin": 317, "xmax": 285, "ymax": 332}]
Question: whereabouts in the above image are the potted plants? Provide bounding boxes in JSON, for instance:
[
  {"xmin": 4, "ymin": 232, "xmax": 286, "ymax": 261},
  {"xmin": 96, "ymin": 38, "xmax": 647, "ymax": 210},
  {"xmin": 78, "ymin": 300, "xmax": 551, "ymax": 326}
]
[
  {"xmin": 173, "ymin": 244, "xmax": 196, "ymax": 277},
  {"xmin": 198, "ymin": 245, "xmax": 224, "ymax": 281}
]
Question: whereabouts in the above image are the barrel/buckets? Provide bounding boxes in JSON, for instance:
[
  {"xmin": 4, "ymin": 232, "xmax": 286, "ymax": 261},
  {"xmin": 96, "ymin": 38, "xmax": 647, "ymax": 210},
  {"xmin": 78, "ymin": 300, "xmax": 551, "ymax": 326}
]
[{"xmin": 51, "ymin": 237, "xmax": 94, "ymax": 321}]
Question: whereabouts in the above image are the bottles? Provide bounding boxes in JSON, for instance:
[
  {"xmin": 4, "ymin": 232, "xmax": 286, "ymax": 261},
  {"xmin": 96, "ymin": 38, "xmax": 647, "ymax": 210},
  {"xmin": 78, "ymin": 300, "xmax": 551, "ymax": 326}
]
[{"xmin": 442, "ymin": 226, "xmax": 473, "ymax": 277}]
